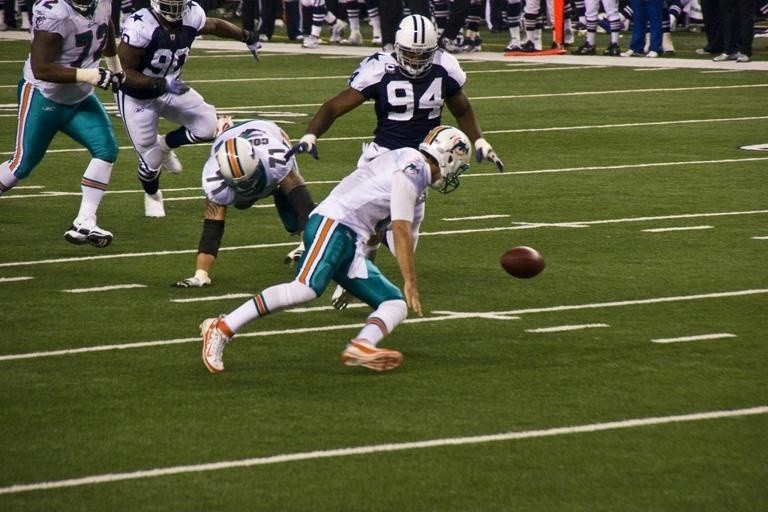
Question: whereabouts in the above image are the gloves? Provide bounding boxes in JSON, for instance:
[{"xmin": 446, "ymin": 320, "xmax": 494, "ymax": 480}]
[
  {"xmin": 475, "ymin": 138, "xmax": 507, "ymax": 174},
  {"xmin": 283, "ymin": 241, "xmax": 306, "ymax": 265},
  {"xmin": 171, "ymin": 268, "xmax": 213, "ymax": 288},
  {"xmin": 242, "ymin": 30, "xmax": 269, "ymax": 62},
  {"xmin": 283, "ymin": 134, "xmax": 321, "ymax": 163},
  {"xmin": 152, "ymin": 67, "xmax": 190, "ymax": 96},
  {"xmin": 76, "ymin": 66, "xmax": 113, "ymax": 91},
  {"xmin": 108, "ymin": 69, "xmax": 128, "ymax": 93}
]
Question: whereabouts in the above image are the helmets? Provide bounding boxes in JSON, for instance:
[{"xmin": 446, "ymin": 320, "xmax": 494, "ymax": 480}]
[
  {"xmin": 215, "ymin": 135, "xmax": 265, "ymax": 199},
  {"xmin": 150, "ymin": 0, "xmax": 192, "ymax": 22},
  {"xmin": 393, "ymin": 12, "xmax": 439, "ymax": 79},
  {"xmin": 417, "ymin": 123, "xmax": 473, "ymax": 194},
  {"xmin": 64, "ymin": 0, "xmax": 98, "ymax": 14}
]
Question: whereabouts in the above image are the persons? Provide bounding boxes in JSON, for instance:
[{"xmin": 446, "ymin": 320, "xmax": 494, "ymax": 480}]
[
  {"xmin": 284, "ymin": 13, "xmax": 503, "ymax": 311},
  {"xmin": 0, "ymin": 0, "xmax": 127, "ymax": 248},
  {"xmin": 0, "ymin": 0, "xmax": 32, "ymax": 31},
  {"xmin": 171, "ymin": 121, "xmax": 316, "ymax": 289},
  {"xmin": 199, "ymin": 124, "xmax": 473, "ymax": 375},
  {"xmin": 437, "ymin": 1, "xmax": 768, "ymax": 62},
  {"xmin": 112, "ymin": 1, "xmax": 263, "ymax": 218},
  {"xmin": 241, "ymin": 0, "xmax": 432, "ymax": 49}
]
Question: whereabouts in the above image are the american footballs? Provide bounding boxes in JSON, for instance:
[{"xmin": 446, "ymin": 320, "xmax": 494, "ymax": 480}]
[{"xmin": 499, "ymin": 245, "xmax": 543, "ymax": 279}]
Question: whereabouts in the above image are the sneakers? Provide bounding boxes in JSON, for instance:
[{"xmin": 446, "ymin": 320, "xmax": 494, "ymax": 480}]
[
  {"xmin": 736, "ymin": 54, "xmax": 752, "ymax": 63},
  {"xmin": 619, "ymin": 48, "xmax": 637, "ymax": 57},
  {"xmin": 603, "ymin": 45, "xmax": 621, "ymax": 56},
  {"xmin": 339, "ymin": 30, "xmax": 364, "ymax": 46},
  {"xmin": 144, "ymin": 189, "xmax": 166, "ymax": 218},
  {"xmin": 571, "ymin": 42, "xmax": 596, "ymax": 55},
  {"xmin": 331, "ymin": 282, "xmax": 352, "ymax": 310},
  {"xmin": 63, "ymin": 221, "xmax": 113, "ymax": 248},
  {"xmin": 157, "ymin": 133, "xmax": 183, "ymax": 174},
  {"xmin": 712, "ymin": 52, "xmax": 730, "ymax": 62},
  {"xmin": 328, "ymin": 18, "xmax": 348, "ymax": 42},
  {"xmin": 563, "ymin": 37, "xmax": 575, "ymax": 47},
  {"xmin": 301, "ymin": 35, "xmax": 319, "ymax": 49},
  {"xmin": 370, "ymin": 34, "xmax": 383, "ymax": 45},
  {"xmin": 459, "ymin": 41, "xmax": 471, "ymax": 52},
  {"xmin": 504, "ymin": 41, "xmax": 520, "ymax": 52},
  {"xmin": 256, "ymin": 33, "xmax": 269, "ymax": 43},
  {"xmin": 695, "ymin": 47, "xmax": 711, "ymax": 56},
  {"xmin": 296, "ymin": 33, "xmax": 303, "ymax": 41},
  {"xmin": 646, "ymin": 50, "xmax": 658, "ymax": 58},
  {"xmin": 520, "ymin": 40, "xmax": 536, "ymax": 52},
  {"xmin": 341, "ymin": 338, "xmax": 404, "ymax": 372},
  {"xmin": 198, "ymin": 314, "xmax": 230, "ymax": 373},
  {"xmin": 471, "ymin": 39, "xmax": 484, "ymax": 53}
]
